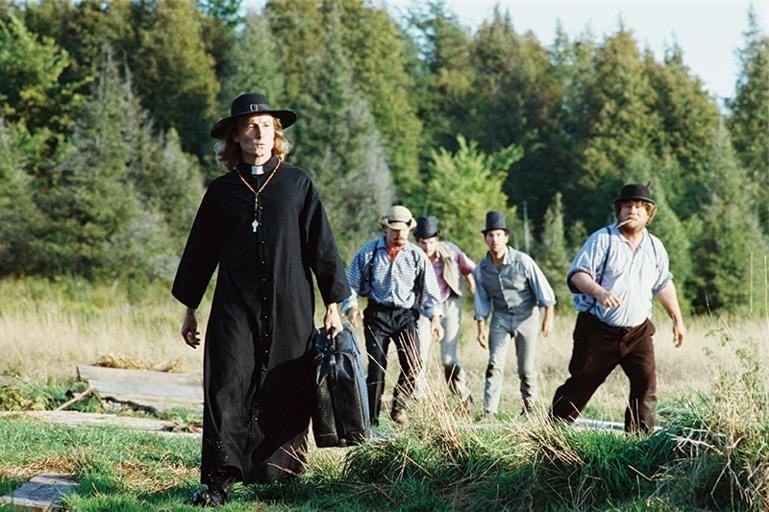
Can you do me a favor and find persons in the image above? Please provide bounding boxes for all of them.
[409,216,480,402]
[340,206,446,426]
[170,92,353,505]
[545,183,685,442]
[473,229,557,423]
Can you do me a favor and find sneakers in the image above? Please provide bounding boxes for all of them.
[190,482,230,509]
[389,406,411,428]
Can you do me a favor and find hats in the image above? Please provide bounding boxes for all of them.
[413,216,440,239]
[481,211,511,234]
[613,183,655,205]
[210,93,296,140]
[379,205,417,230]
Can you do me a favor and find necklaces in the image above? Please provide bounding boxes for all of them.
[237,158,282,232]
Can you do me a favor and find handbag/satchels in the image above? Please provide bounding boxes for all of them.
[358,271,371,297]
[312,322,370,449]
